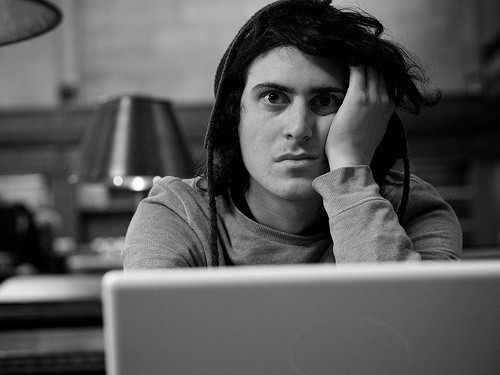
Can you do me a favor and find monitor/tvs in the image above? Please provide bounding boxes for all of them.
[102,265,500,375]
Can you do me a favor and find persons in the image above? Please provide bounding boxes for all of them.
[121,1,463,272]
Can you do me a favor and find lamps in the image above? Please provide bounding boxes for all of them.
[77,93,199,190]
